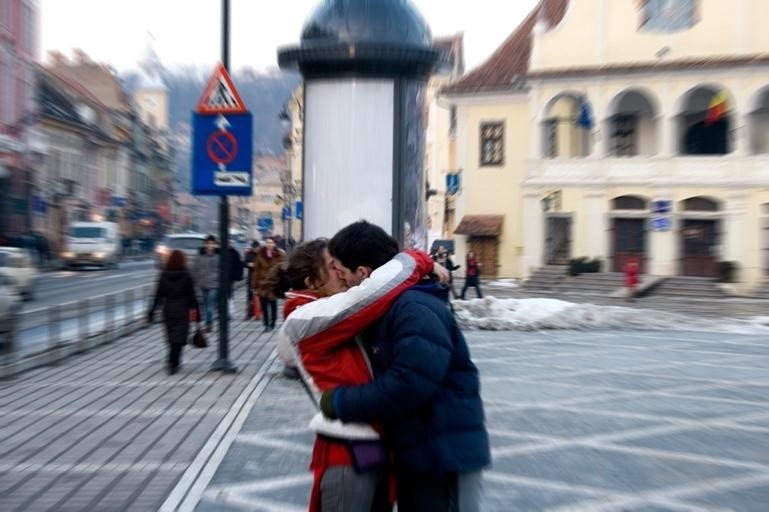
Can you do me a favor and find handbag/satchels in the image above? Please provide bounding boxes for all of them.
[192,321,210,349]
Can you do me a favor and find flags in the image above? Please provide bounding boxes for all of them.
[703,87,732,125]
[571,98,593,129]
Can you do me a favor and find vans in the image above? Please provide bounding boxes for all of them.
[59,222,122,272]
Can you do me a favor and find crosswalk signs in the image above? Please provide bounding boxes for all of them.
[195,64,249,117]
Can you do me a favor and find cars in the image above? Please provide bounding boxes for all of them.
[0,244,42,319]
[155,227,247,271]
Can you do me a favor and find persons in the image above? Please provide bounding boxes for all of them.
[262,235,450,512]
[315,220,493,512]
